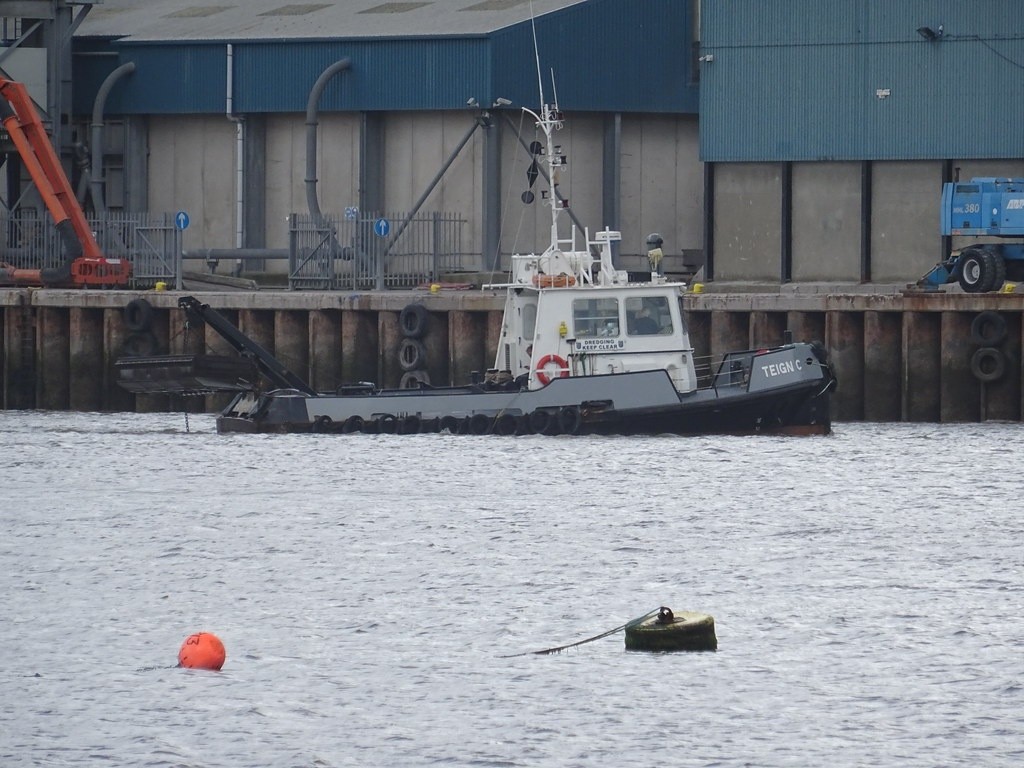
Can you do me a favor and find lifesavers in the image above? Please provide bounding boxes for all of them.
[533,275,575,287]
[309,405,582,437]
[969,347,1006,383]
[536,354,570,386]
[970,310,1009,348]
[395,303,429,391]
[124,297,157,356]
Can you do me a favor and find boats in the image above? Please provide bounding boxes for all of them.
[113,10,844,445]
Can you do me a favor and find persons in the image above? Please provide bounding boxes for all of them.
[632,307,664,335]
